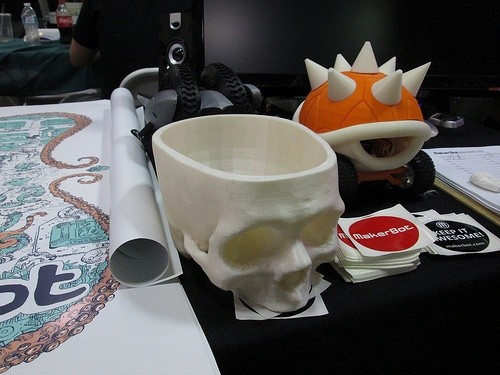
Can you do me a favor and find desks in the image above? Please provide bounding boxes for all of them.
[0,39,92,96]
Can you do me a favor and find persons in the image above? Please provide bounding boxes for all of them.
[69,0,204,99]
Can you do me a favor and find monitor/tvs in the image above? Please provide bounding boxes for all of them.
[193,0,500,98]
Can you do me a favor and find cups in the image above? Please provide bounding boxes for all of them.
[0,13,13,42]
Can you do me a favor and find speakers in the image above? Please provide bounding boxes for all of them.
[158,12,197,91]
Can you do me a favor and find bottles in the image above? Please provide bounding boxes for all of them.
[55,0,73,43]
[21,3,41,46]
